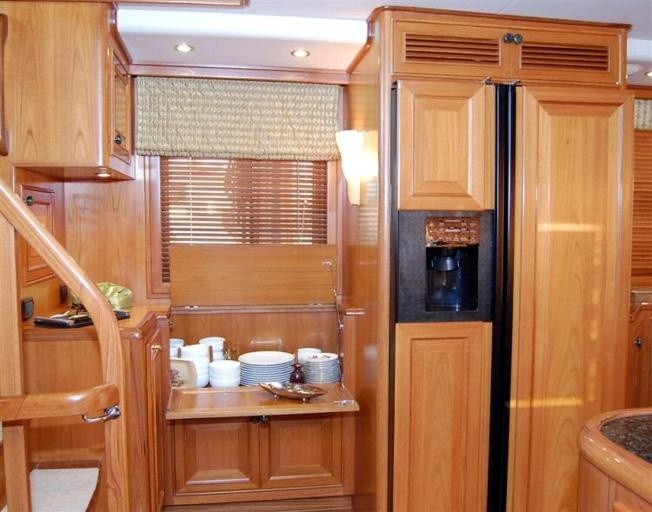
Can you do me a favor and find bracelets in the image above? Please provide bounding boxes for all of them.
[169,334,241,390]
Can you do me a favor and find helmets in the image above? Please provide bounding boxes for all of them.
[297,347,321,362]
[237,350,297,386]
[302,352,339,386]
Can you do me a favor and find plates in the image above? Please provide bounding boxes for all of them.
[28,317,164,512]
[175,417,347,493]
[5,1,136,179]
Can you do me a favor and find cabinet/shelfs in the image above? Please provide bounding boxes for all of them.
[175,417,347,493]
[28,317,164,512]
[289,363,307,384]
[5,1,136,179]
[347,34,626,510]
